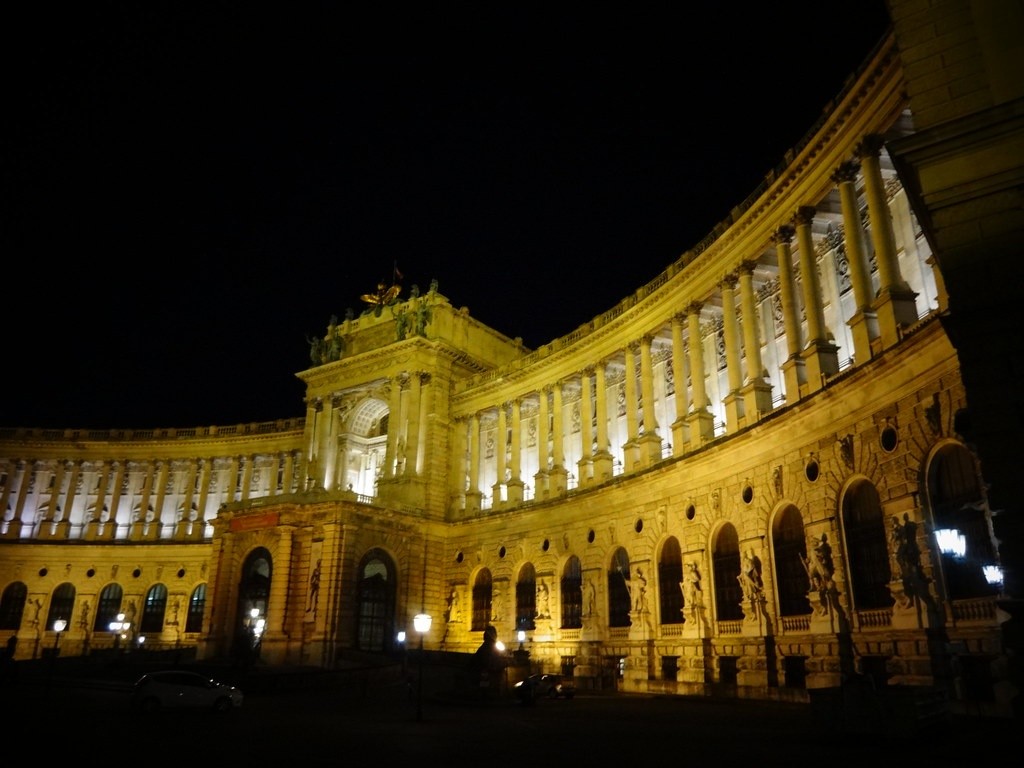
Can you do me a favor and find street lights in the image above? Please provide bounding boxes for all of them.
[108,612,136,651]
[53,617,67,649]
[413,613,432,722]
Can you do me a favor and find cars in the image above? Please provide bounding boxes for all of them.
[513,672,577,700]
[130,670,247,711]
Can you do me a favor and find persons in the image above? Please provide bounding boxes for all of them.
[888,516,914,579]
[681,561,699,608]
[491,590,504,620]
[448,592,461,622]
[77,600,91,627]
[307,557,321,613]
[805,537,831,591]
[627,570,649,612]
[121,600,135,627]
[327,330,342,363]
[394,310,409,341]
[581,579,595,615]
[309,336,324,369]
[28,598,41,623]
[736,551,760,604]
[535,584,549,619]
[415,304,428,339]
[166,594,180,625]
